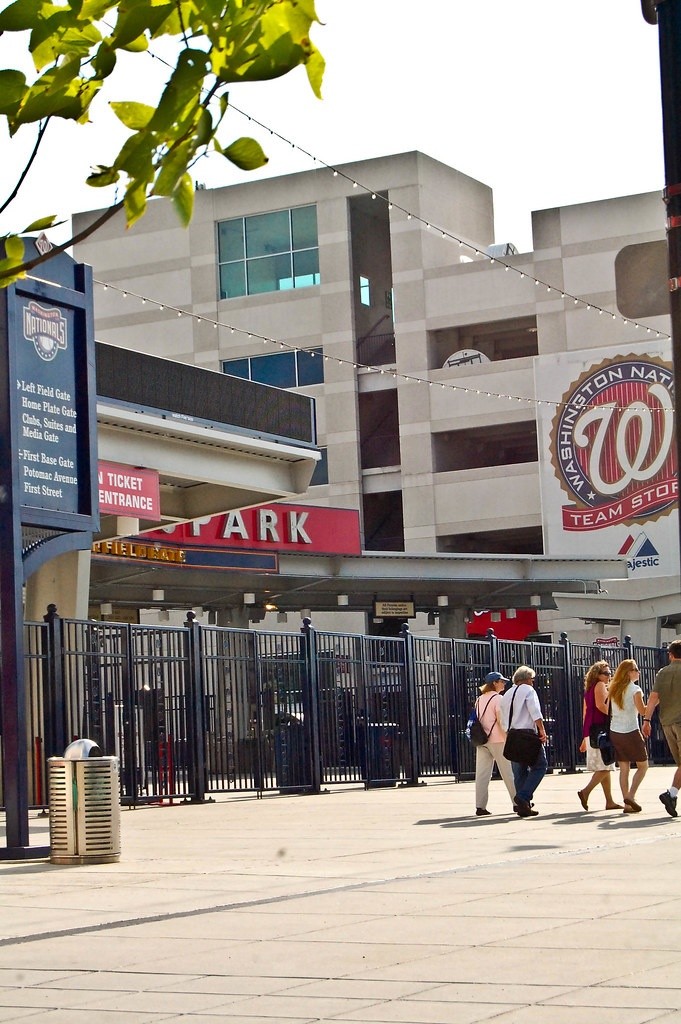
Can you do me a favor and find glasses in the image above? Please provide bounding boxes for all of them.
[629,667,639,673]
[598,671,612,676]
[496,680,506,684]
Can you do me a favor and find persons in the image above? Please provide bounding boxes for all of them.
[472,672,534,815]
[640,640,681,817]
[500,666,548,817]
[576,660,625,810]
[604,656,660,813]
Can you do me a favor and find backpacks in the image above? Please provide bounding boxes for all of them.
[465,694,498,745]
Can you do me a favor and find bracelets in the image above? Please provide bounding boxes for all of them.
[643,718,650,721]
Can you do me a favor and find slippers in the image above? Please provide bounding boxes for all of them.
[606,804,625,809]
[577,790,588,811]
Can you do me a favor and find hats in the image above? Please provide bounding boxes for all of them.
[485,672,511,683]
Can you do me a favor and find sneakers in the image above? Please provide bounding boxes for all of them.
[659,789,678,817]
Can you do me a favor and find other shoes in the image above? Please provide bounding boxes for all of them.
[623,795,642,812]
[624,807,639,813]
[513,803,535,812]
[476,807,491,815]
[517,802,539,817]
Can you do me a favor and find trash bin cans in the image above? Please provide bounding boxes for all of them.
[47,738,122,866]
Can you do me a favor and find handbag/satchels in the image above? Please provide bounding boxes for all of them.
[598,733,616,766]
[588,723,610,748]
[503,729,542,766]
